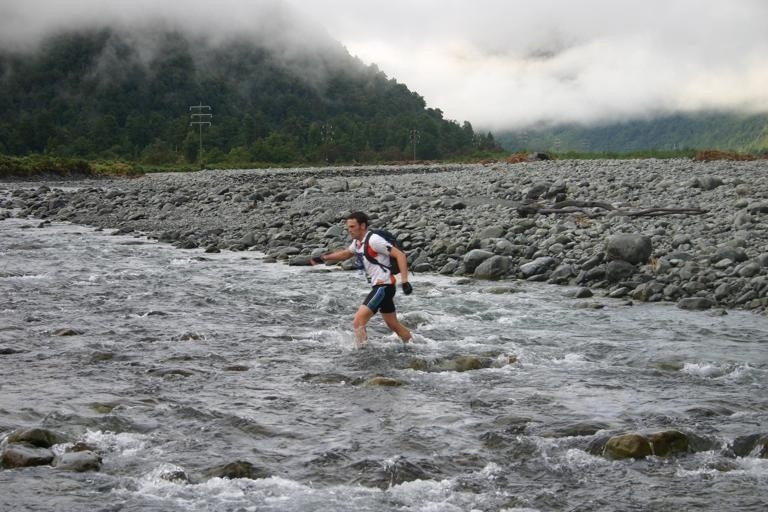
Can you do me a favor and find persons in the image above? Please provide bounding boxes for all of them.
[307,211,413,344]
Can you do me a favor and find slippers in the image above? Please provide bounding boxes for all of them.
[364,229,403,275]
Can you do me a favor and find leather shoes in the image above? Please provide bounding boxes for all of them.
[313,257,324,264]
[402,282,416,295]
